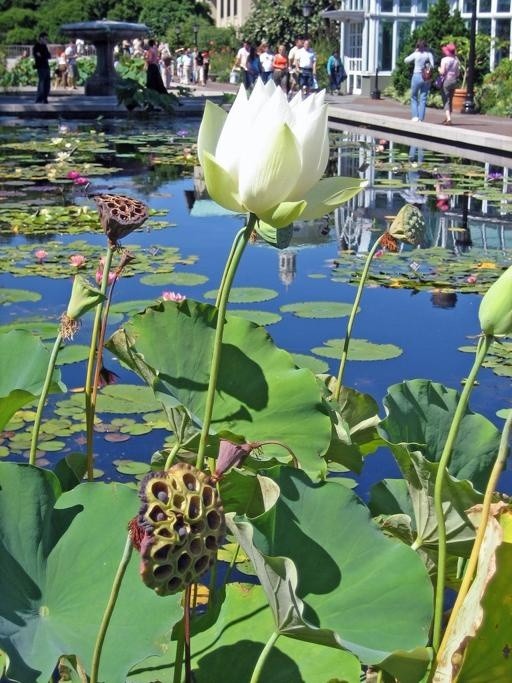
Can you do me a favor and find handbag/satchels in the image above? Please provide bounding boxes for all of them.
[432,74,445,89]
[310,76,318,90]
[422,62,433,80]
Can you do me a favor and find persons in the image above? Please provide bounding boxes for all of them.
[18,48,30,59]
[403,39,436,122]
[235,33,317,102]
[327,46,347,96]
[51,36,212,91]
[436,42,461,125]
[33,31,51,104]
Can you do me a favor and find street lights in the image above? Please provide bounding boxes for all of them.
[174,23,182,49]
[452,158,474,256]
[298,0,317,40]
[192,21,200,47]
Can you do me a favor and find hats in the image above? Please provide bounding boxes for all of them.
[441,44,455,56]
